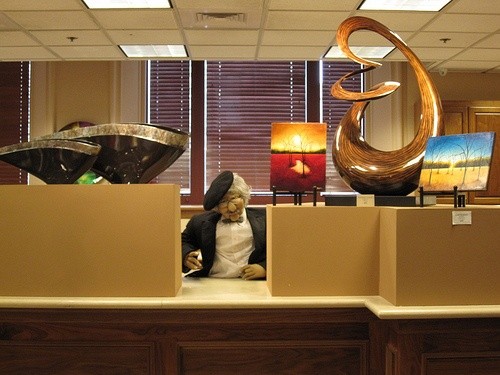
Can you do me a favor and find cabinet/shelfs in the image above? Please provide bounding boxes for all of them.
[414,100,500,205]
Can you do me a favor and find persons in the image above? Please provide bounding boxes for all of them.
[181,171,267,281]
